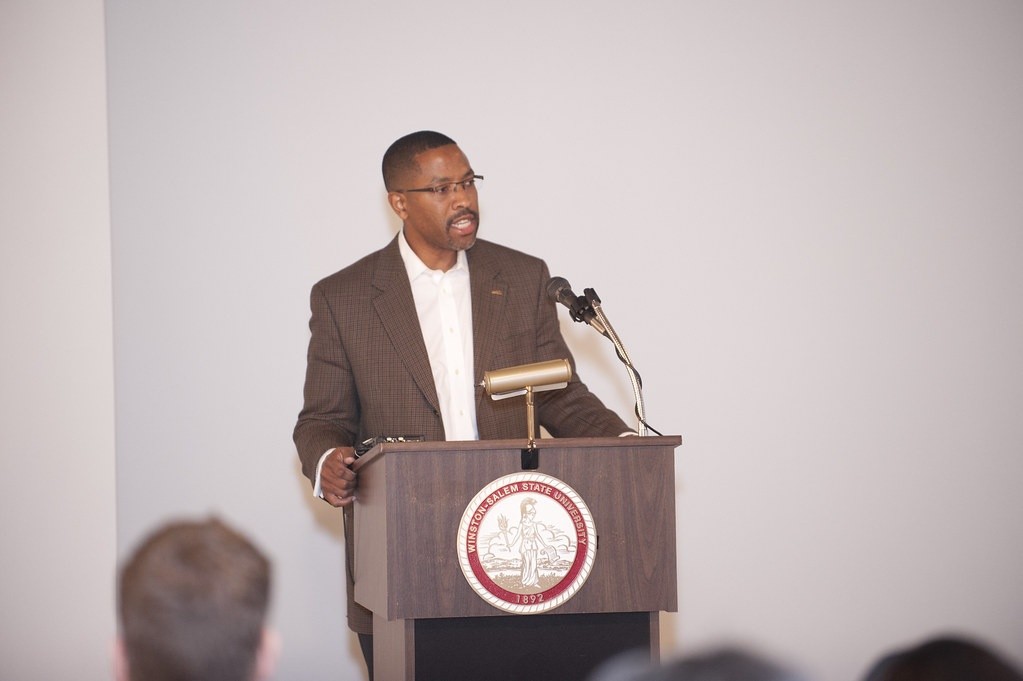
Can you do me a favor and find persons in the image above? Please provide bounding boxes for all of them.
[116,519,275,681]
[594,634,1023,681]
[293,130,638,681]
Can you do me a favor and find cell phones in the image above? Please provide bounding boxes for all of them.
[363,436,426,450]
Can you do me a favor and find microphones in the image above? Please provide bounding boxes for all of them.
[547,277,610,339]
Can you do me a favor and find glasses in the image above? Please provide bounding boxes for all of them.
[389,174,484,198]
